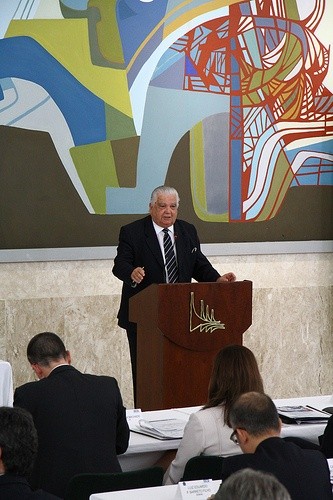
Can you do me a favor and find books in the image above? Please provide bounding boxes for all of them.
[126,408,190,440]
[275,404,332,425]
[174,479,221,500]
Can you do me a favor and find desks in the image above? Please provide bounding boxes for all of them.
[119,395,333,456]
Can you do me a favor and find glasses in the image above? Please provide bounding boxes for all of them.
[230,430,239,446]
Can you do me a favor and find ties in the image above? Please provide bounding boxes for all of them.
[162,228,179,284]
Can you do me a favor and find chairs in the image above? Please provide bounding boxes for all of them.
[72,466,164,500]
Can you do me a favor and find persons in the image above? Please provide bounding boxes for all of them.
[0,405,87,500]
[207,468,291,500]
[13,332,130,475]
[318,413,333,459]
[112,185,236,409]
[219,391,333,500]
[161,343,265,487]
